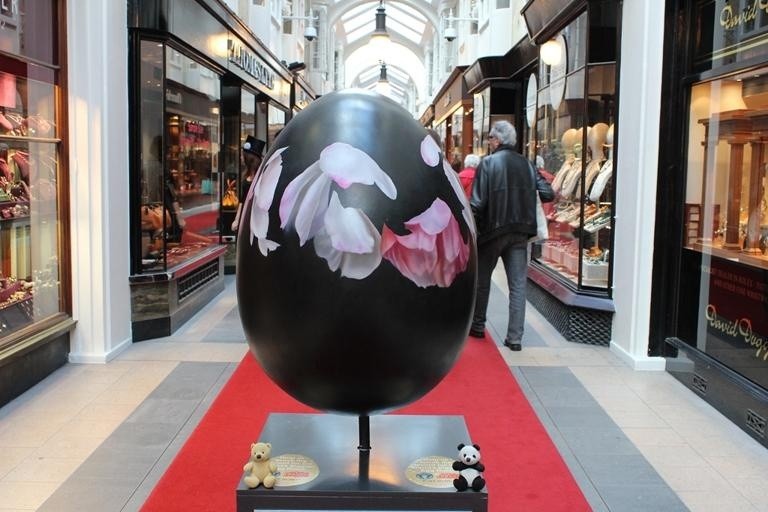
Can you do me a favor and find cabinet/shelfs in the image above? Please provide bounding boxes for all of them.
[0,57,65,358]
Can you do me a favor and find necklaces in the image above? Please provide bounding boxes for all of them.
[0,109,59,218]
[545,160,614,226]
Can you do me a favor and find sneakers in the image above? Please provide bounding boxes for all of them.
[503,339,521,351]
[469,328,485,338]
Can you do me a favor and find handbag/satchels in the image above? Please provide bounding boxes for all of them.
[142,206,172,231]
[531,189,549,244]
[222,179,241,209]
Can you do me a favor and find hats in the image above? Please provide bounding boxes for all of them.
[240,135,266,157]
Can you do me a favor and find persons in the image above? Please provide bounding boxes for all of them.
[147,136,185,246]
[458,153,481,200]
[469,120,554,352]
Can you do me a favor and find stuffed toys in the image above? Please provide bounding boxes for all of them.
[243,442,278,489]
[451,443,486,491]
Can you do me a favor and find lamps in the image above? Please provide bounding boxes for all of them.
[440,7,481,42]
[357,0,399,94]
[283,7,319,42]
